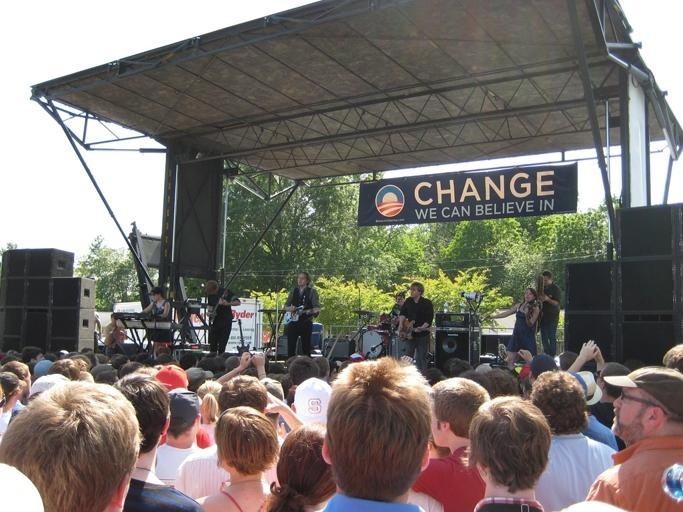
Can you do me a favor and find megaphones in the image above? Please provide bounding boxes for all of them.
[461,291,481,303]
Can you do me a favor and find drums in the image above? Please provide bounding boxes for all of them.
[355,331,384,359]
[378,329,389,348]
[368,325,377,331]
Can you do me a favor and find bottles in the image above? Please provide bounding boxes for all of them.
[443,302,448,313]
[661,463,683,502]
[460,301,465,312]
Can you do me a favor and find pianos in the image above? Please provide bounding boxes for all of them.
[111,313,168,322]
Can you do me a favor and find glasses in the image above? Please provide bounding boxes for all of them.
[619,392,669,417]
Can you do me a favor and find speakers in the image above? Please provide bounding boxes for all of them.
[0,248,95,353]
[435,312,481,368]
[324,336,355,358]
[565,202,683,368]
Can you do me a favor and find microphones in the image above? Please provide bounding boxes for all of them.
[146,292,154,296]
[371,340,384,351]
[293,305,304,312]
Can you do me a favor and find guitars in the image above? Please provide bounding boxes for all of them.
[207,291,228,318]
[398,318,433,338]
[284,305,319,325]
[237,319,249,356]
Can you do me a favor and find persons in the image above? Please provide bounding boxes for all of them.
[408,340,683,512]
[94,312,118,356]
[392,281,434,370]
[488,288,541,370]
[1,347,364,512]
[143,287,172,358]
[539,272,561,358]
[285,273,319,356]
[207,280,241,355]
[322,357,432,512]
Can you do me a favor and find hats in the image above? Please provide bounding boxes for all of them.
[155,364,213,423]
[295,376,334,437]
[603,366,683,418]
[29,374,70,397]
[576,371,602,406]
[33,360,53,376]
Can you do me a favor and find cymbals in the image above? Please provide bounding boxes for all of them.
[351,311,371,315]
